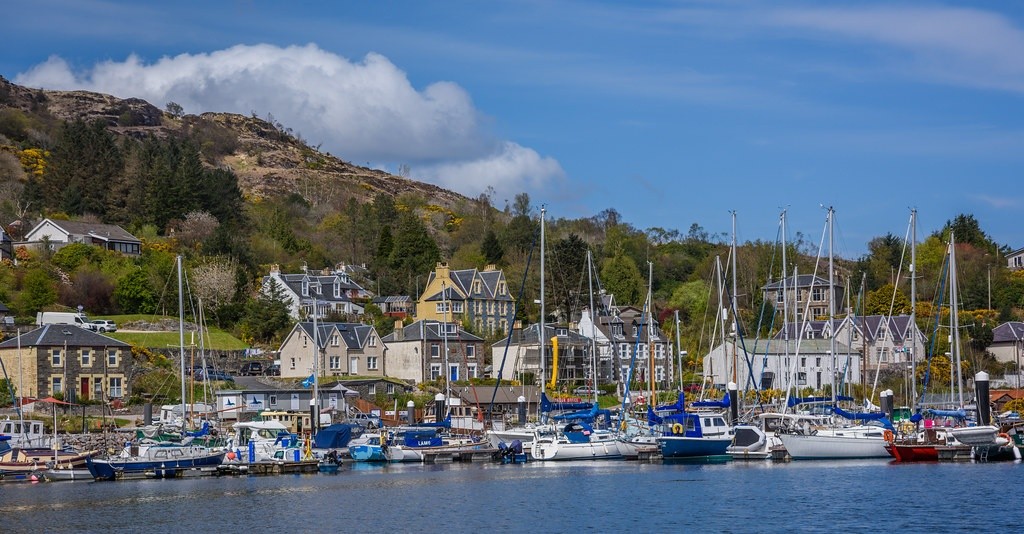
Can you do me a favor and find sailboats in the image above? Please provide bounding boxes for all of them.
[86,255,227,480]
[863,206,920,415]
[484,203,570,449]
[609,256,772,461]
[0,358,102,481]
[890,227,1024,444]
[724,206,892,463]
[529,249,638,462]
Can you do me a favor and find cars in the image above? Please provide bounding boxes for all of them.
[263,365,280,376]
[561,385,606,396]
[2,317,14,323]
[186,365,235,383]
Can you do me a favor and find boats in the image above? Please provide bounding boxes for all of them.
[883,428,971,463]
[306,281,501,464]
[973,433,1024,463]
[217,420,319,474]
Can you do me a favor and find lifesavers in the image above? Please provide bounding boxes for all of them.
[903,422,913,434]
[998,433,1010,446]
[637,396,644,405]
[673,423,683,434]
[856,419,860,423]
[884,430,893,442]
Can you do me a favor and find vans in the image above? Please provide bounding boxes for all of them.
[36,311,95,331]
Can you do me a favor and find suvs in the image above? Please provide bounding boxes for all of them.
[683,383,725,394]
[90,320,117,333]
[240,362,262,376]
